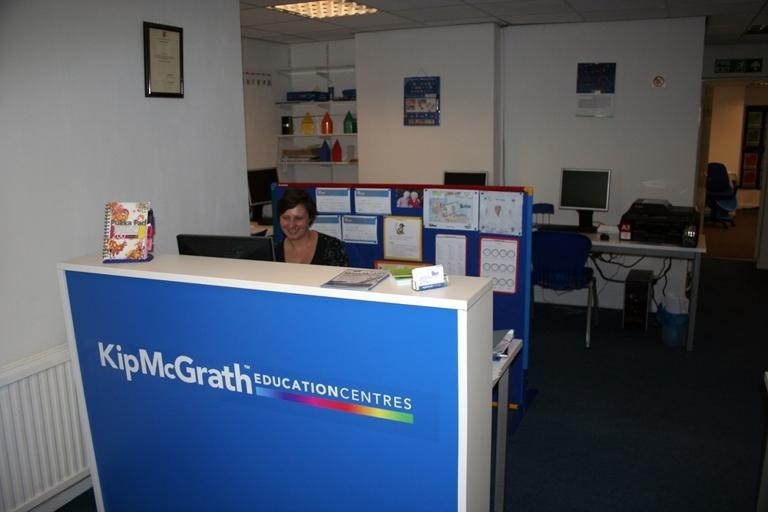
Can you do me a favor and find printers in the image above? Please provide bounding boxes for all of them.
[619,198,701,248]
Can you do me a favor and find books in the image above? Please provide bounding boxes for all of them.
[321,269,388,290]
[100,200,155,263]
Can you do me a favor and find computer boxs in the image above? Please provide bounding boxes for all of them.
[622,268,654,333]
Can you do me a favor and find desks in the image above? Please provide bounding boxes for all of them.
[580,224,707,352]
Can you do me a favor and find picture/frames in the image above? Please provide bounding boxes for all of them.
[142,21,185,99]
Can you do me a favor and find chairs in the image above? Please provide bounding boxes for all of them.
[530,229,599,349]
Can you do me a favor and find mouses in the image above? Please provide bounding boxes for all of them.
[600,232,610,240]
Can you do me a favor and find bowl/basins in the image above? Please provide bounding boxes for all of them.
[342,89,356,98]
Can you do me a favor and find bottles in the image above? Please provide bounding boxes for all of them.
[281,111,357,163]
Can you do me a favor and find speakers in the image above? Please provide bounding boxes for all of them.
[281,116,293,134]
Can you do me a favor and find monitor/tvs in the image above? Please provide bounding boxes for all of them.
[247,168,280,221]
[176,234,277,261]
[558,167,612,233]
[443,171,487,186]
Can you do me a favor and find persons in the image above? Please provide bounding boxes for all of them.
[268,189,347,267]
[396,190,423,210]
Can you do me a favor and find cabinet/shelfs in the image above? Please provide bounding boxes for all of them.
[274,97,359,166]
